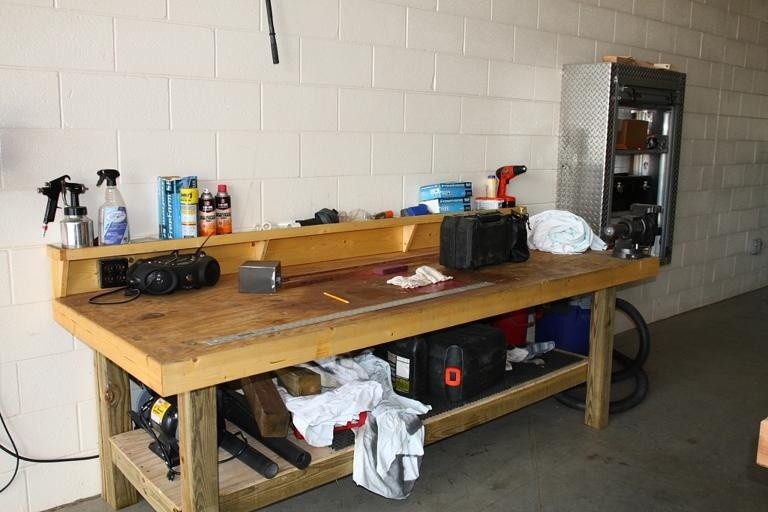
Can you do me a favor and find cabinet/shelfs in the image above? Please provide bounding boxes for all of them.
[555,62,686,266]
[47,206,660,512]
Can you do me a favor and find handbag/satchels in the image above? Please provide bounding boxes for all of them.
[436,211,528,270]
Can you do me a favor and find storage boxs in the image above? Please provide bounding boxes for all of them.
[428,324,507,402]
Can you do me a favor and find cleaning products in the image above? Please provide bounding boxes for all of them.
[96,168,131,246]
[34,175,95,248]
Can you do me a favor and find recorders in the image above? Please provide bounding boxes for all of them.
[126,253,222,296]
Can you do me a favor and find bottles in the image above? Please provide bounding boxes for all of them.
[180,184,232,239]
[487,175,498,198]
